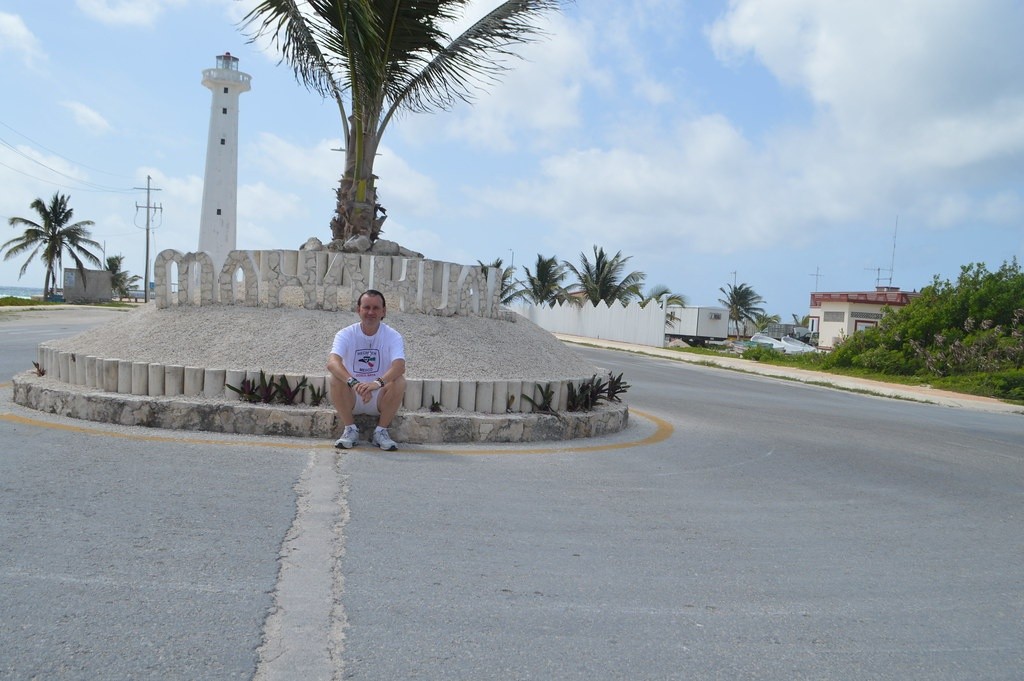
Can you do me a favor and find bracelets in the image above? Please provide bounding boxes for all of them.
[374,376,387,388]
[350,380,360,389]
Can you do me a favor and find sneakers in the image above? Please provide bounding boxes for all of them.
[371,428,399,451]
[334,426,359,449]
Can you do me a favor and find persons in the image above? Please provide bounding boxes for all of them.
[326,289,408,450]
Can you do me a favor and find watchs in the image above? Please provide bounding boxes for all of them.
[347,377,357,388]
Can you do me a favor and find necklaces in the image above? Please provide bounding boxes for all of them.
[360,321,380,357]
